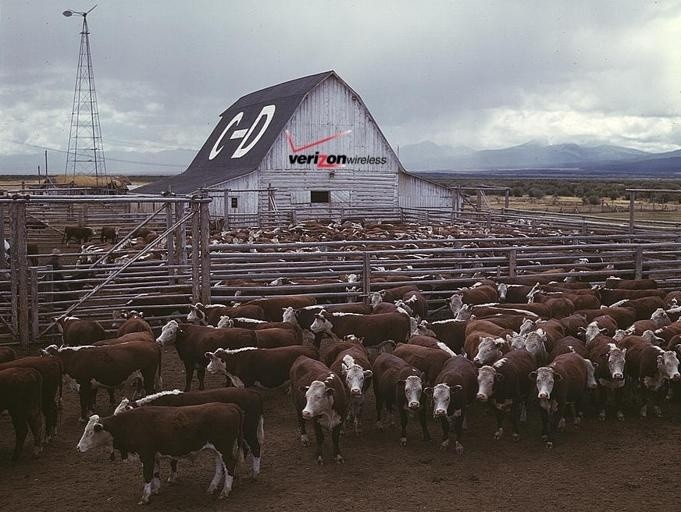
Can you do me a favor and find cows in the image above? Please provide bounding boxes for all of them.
[0,216,680,504]
